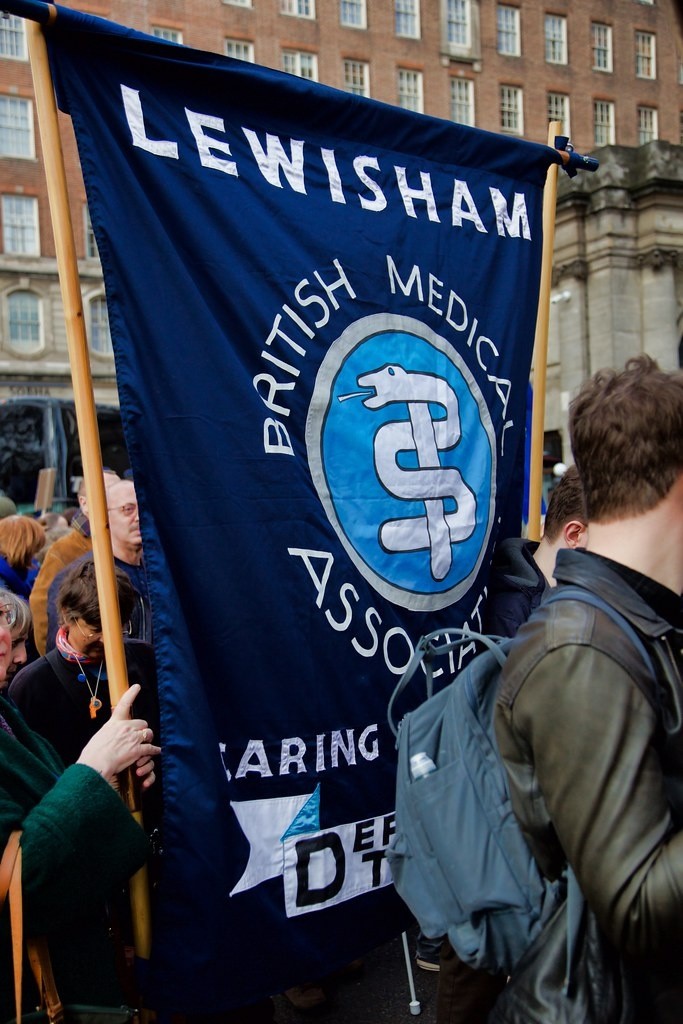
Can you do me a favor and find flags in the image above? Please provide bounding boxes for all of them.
[0,0,558,1019]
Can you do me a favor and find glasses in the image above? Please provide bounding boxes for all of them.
[0,603,18,629]
[72,614,132,641]
[105,503,135,514]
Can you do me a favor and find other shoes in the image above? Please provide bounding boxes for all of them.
[337,959,363,973]
[415,953,440,971]
[284,985,326,1009]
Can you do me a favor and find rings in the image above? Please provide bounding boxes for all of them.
[140,729,147,743]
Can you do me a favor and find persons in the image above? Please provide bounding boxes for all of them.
[0,353,683,1024]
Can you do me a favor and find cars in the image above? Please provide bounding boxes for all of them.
[1,396,129,504]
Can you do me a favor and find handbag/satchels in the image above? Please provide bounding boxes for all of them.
[485,873,637,1024]
[0,832,142,1024]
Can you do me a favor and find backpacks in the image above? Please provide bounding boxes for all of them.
[387,589,662,974]
